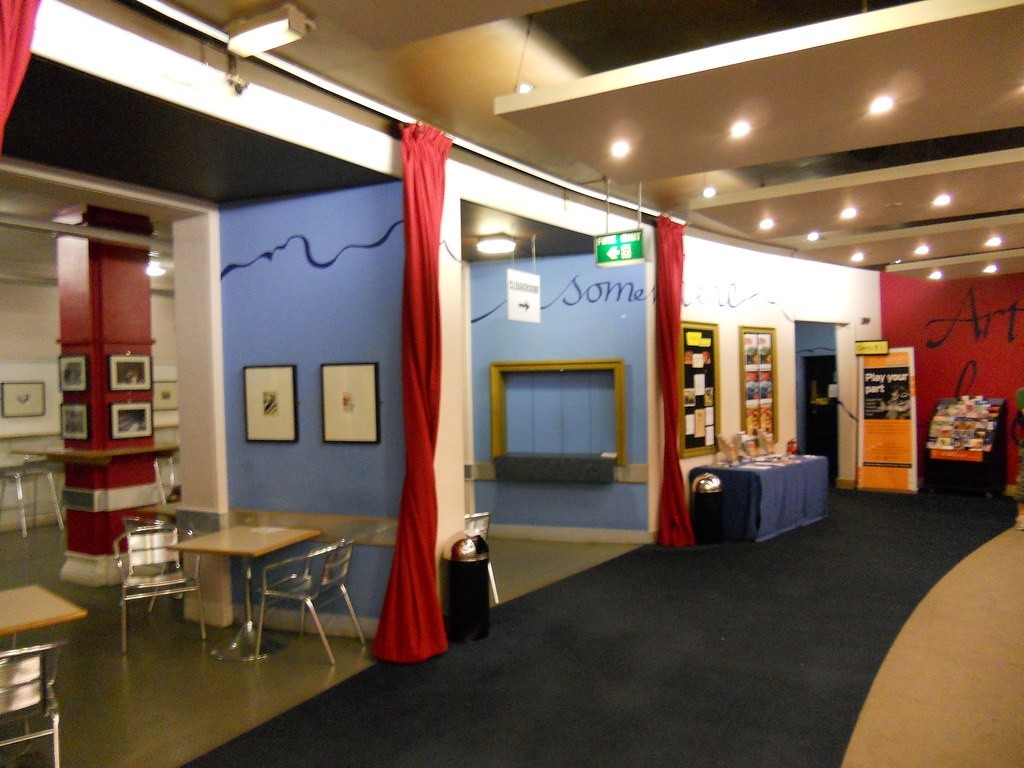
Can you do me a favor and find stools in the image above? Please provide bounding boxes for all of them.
[0,453,64,536]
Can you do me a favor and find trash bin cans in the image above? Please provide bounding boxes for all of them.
[441,531,493,644]
[692,473,726,548]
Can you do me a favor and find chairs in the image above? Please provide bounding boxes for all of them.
[112,517,206,655]
[464,512,500,604]
[0,640,70,768]
[255,536,366,667]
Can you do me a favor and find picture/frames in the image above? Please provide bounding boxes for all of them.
[1,383,46,417]
[60,403,89,440]
[109,401,154,441]
[153,381,178,411]
[243,364,300,443]
[58,354,88,393]
[738,326,779,446]
[677,321,722,458]
[108,353,154,392]
[320,363,381,444]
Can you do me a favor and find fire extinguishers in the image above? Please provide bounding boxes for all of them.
[788,439,798,453]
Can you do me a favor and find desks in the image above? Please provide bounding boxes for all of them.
[0,583,89,764]
[689,454,830,542]
[166,526,323,662]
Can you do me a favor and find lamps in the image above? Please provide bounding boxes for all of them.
[474,233,517,255]
[227,4,306,58]
